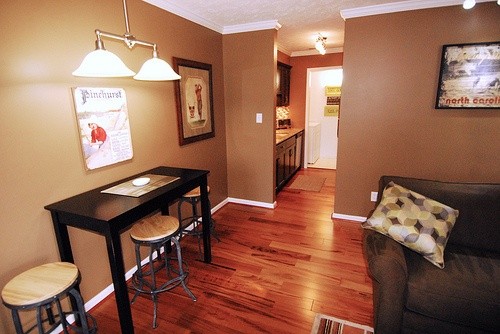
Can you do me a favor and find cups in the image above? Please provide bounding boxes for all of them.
[187,101,196,118]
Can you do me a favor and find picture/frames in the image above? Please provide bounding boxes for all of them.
[172,56,215,146]
[435,41,500,109]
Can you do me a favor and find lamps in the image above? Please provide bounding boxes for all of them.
[315,33,327,55]
[71,0,182,81]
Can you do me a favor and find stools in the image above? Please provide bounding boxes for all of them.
[178,185,220,256]
[130,216,197,328]
[2,262,97,334]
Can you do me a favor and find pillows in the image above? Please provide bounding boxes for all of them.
[361,181,459,269]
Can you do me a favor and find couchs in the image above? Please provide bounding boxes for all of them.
[364,176,500,334]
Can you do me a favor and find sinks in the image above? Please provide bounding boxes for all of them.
[276,133,288,142]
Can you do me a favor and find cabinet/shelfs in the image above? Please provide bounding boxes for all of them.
[276,61,304,194]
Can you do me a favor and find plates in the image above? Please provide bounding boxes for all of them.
[132,176,151,186]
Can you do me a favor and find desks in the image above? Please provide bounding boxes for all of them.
[43,166,210,334]
